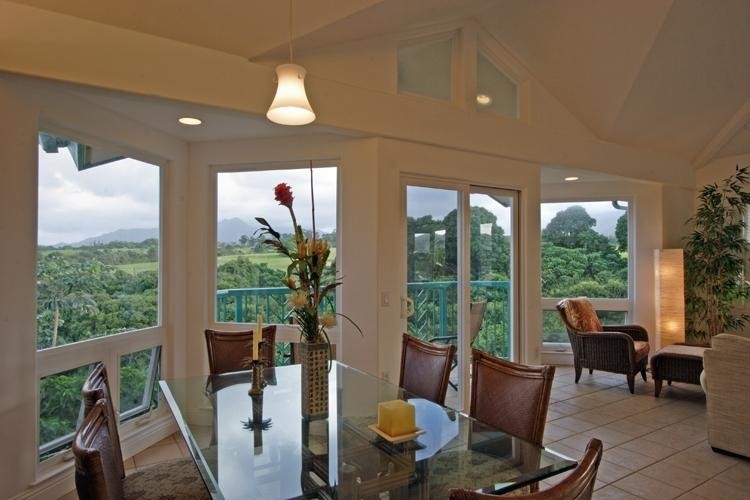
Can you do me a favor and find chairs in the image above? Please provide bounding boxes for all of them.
[204,323,280,446]
[447,438,603,500]
[700,334,750,463]
[72,362,213,499]
[415,347,557,499]
[342,332,455,457]
[556,296,650,394]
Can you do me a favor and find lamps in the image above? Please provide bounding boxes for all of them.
[266,0,317,125]
[654,249,686,351]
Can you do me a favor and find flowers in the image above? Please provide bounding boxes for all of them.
[253,182,363,372]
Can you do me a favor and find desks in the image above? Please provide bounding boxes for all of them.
[157,359,579,499]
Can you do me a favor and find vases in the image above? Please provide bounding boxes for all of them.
[299,342,330,420]
